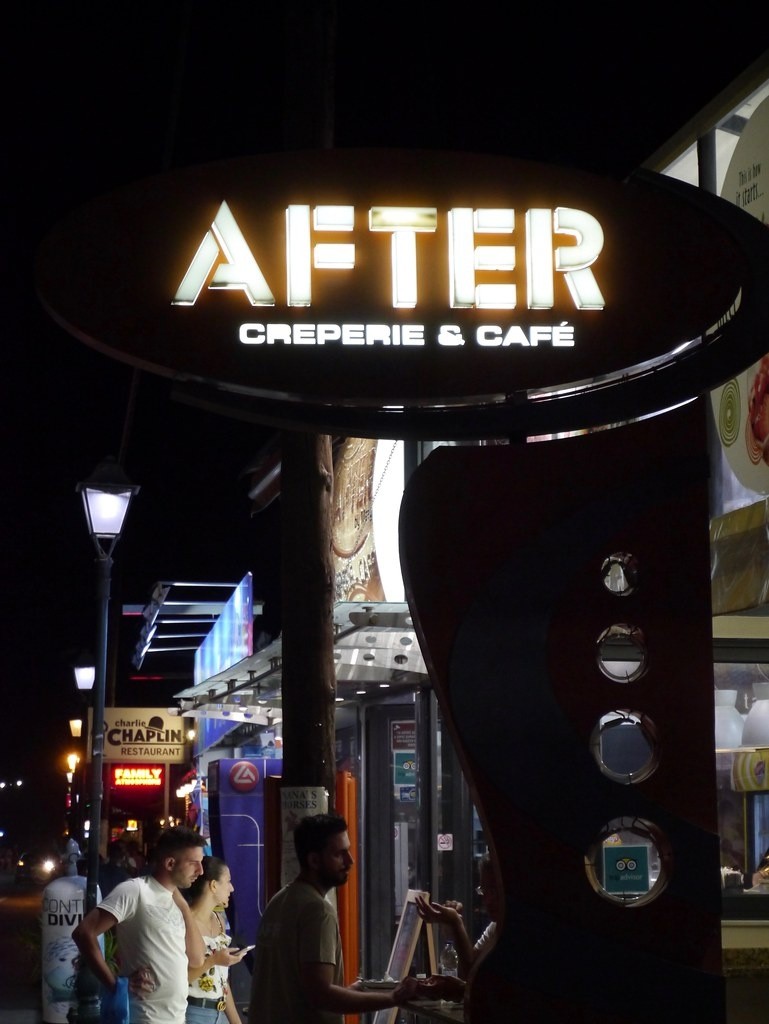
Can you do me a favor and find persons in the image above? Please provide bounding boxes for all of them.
[248,815,419,1024]
[414,854,499,1002]
[71,827,247,1024]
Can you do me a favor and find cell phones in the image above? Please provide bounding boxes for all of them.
[233,945,256,955]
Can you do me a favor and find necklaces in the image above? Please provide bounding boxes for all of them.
[195,911,215,939]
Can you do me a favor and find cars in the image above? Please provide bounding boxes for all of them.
[13,853,49,885]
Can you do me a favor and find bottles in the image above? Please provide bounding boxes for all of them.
[440,938,458,979]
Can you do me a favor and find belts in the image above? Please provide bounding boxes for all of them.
[187,995,226,1011]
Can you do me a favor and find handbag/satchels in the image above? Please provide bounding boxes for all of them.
[101,977,130,1024]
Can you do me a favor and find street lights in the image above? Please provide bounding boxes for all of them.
[67,752,80,839]
[73,454,144,1023]
[72,651,96,873]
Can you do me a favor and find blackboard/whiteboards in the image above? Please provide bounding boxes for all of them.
[369,889,437,1024]
